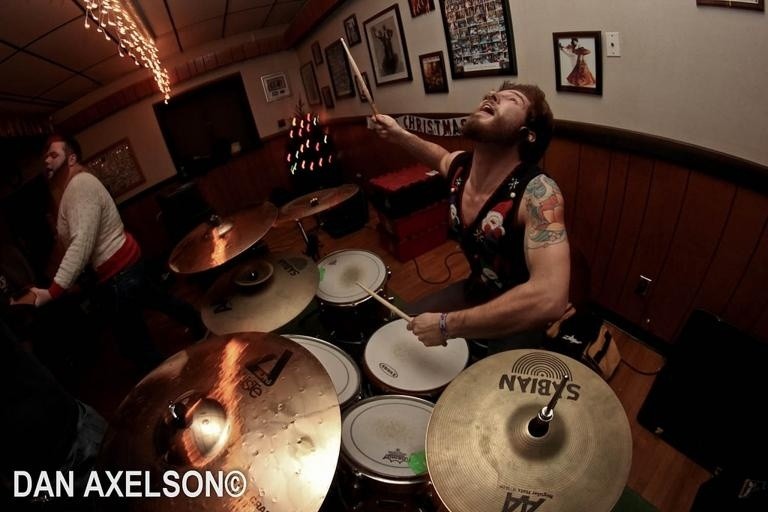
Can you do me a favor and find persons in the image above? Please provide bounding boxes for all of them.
[372,25,398,75]
[28,135,210,376]
[369,80,571,361]
[1,290,107,467]
[558,37,596,87]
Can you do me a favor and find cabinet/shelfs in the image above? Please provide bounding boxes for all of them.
[367,163,450,263]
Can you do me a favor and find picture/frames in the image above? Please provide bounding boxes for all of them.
[81,136,148,200]
[297,1,605,111]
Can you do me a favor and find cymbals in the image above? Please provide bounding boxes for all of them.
[425,348,633,512]
[99,332,342,512]
[169,201,278,275]
[280,183,359,218]
[200,251,320,337]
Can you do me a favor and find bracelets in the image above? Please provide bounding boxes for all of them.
[439,311,448,347]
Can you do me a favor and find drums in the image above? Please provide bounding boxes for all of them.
[317,248,394,345]
[359,314,469,405]
[277,334,363,415]
[340,394,436,512]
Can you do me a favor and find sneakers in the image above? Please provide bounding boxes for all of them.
[189,311,205,336]
